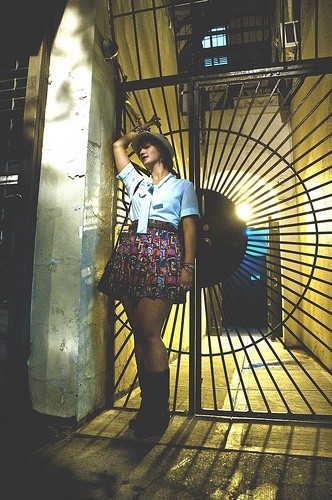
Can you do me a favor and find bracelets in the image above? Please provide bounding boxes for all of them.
[129,132,135,139]
[133,126,143,133]
[183,266,193,275]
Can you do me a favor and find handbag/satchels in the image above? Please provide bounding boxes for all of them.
[97,249,119,300]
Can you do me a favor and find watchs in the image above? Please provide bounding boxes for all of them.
[183,263,195,269]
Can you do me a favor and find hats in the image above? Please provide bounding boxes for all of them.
[132,132,174,161]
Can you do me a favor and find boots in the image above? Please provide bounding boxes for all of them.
[141,368,170,437]
[129,352,147,429]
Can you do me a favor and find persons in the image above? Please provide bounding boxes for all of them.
[98,114,201,440]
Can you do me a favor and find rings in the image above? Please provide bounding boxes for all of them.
[183,286,187,289]
[181,285,184,287]
[187,285,191,288]
